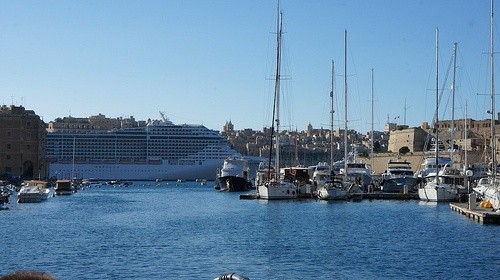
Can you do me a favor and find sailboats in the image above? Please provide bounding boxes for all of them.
[256,1,500,208]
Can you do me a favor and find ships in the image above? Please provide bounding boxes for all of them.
[46,123,270,182]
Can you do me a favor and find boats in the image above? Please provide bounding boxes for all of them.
[0,177,119,210]
[213,153,252,192]
